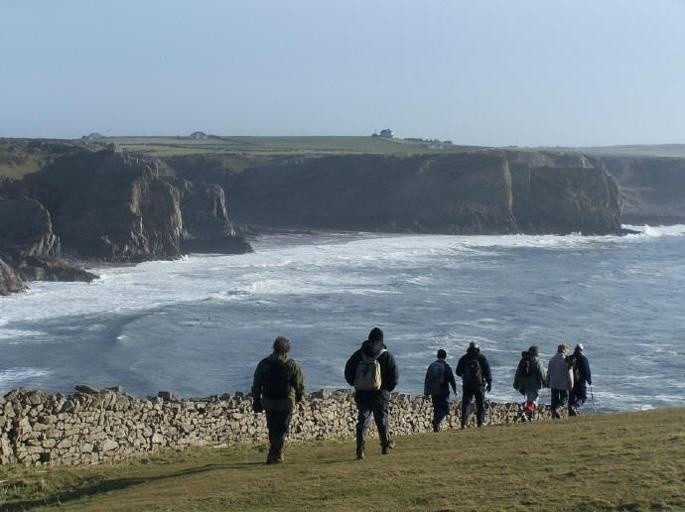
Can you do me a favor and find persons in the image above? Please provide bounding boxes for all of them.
[545,344,572,420]
[513,345,547,422]
[252,335,304,465]
[567,343,592,418]
[422,349,458,433]
[344,327,400,460]
[455,341,493,429]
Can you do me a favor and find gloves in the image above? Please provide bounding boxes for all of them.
[543,384,550,388]
[588,381,592,385]
[454,391,457,397]
[296,395,304,402]
[253,399,264,413]
[486,382,492,392]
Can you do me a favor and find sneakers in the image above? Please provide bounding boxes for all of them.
[521,406,581,421]
[358,452,366,459]
[383,440,395,454]
[266,452,284,464]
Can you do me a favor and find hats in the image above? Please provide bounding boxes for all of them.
[368,327,382,341]
[437,350,446,357]
[470,341,479,348]
[576,343,583,353]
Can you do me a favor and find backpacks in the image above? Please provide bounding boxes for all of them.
[353,347,387,392]
[462,357,483,385]
[426,360,447,396]
[521,354,533,377]
[567,355,580,383]
[264,360,293,401]
